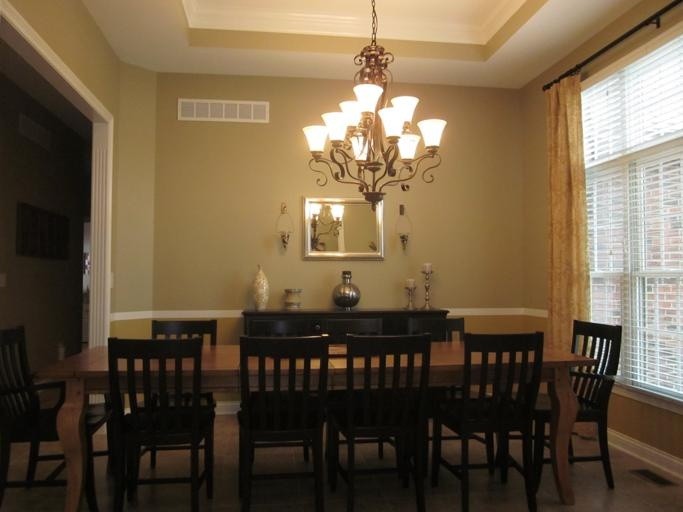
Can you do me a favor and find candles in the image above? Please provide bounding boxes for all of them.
[423,262,432,273]
[406,278,415,287]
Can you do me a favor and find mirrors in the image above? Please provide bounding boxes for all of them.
[300,195,385,261]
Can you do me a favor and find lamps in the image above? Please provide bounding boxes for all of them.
[308,203,345,251]
[301,1,448,215]
[275,202,294,251]
[392,204,413,253]
